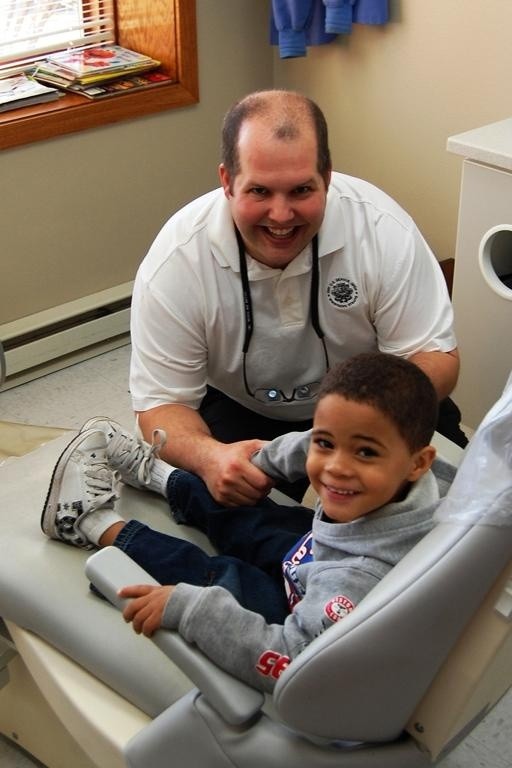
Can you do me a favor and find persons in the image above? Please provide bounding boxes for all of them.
[127,87,462,509]
[36,350,444,750]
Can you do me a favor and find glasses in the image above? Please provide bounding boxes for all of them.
[243,338,331,405]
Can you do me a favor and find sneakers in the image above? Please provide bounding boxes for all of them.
[41,429,116,550]
[80,415,163,492]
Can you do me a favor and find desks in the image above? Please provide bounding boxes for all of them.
[445,117,512,430]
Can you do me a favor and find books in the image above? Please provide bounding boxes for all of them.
[0,43,171,114]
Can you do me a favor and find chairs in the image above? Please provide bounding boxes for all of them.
[0,370,512,768]
